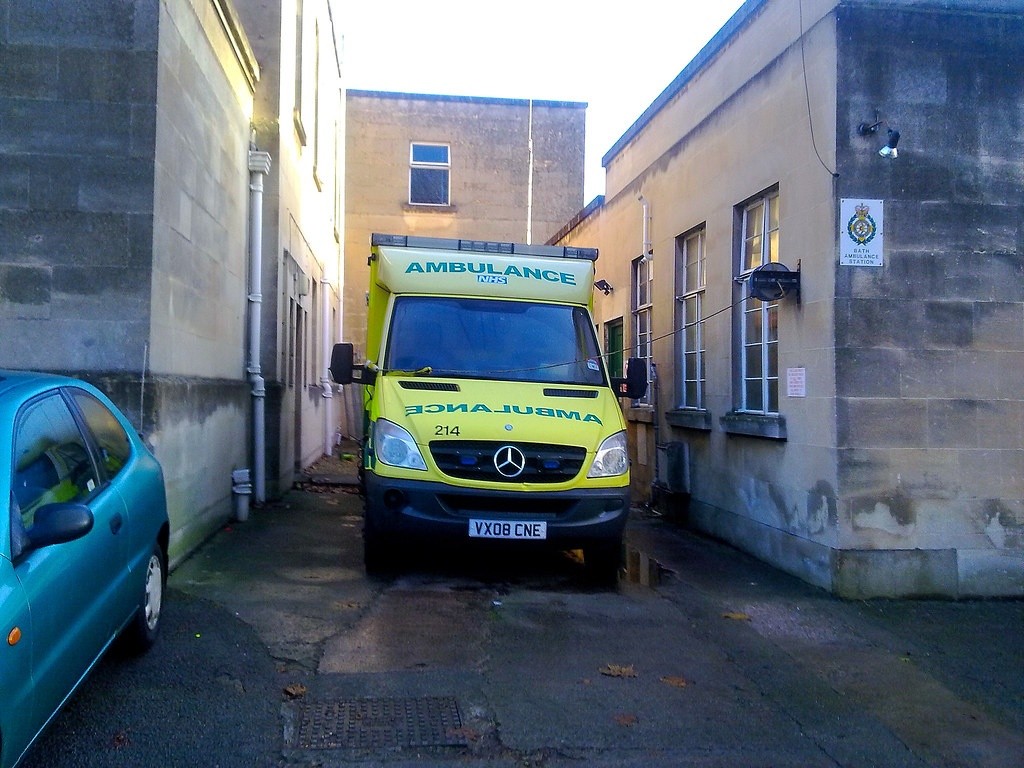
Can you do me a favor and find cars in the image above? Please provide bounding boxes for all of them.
[0,369,171,768]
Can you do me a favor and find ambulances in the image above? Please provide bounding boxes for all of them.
[329,232,653,583]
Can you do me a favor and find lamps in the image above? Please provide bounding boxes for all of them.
[857,107,901,159]
[594,280,613,296]
